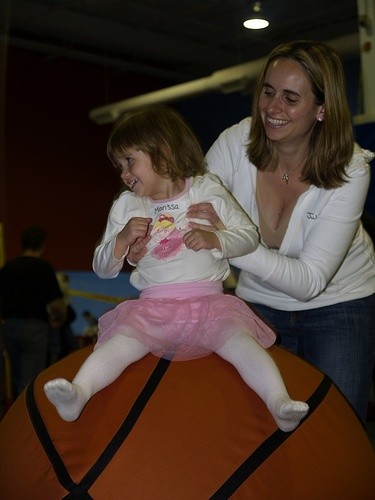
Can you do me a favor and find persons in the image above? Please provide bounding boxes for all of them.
[43,108,309,433]
[0,225,70,404]
[126,41,375,428]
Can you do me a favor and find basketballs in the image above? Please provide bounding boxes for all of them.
[0,341,374,500]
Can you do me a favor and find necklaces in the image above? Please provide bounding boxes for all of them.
[271,147,310,185]
